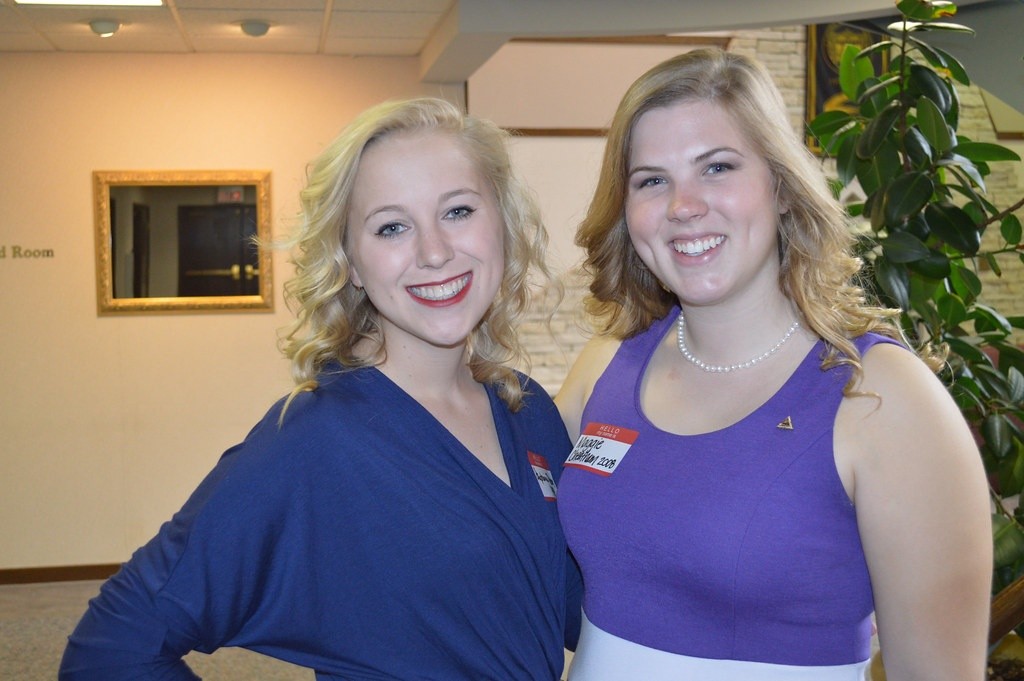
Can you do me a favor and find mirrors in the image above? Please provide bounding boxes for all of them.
[92,168,277,317]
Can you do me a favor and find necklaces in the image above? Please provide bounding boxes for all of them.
[676,311,800,374]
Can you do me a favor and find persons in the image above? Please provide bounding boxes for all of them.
[554,47,993,681]
[57,96,583,681]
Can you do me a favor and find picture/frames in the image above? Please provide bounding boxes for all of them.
[806,20,893,159]
